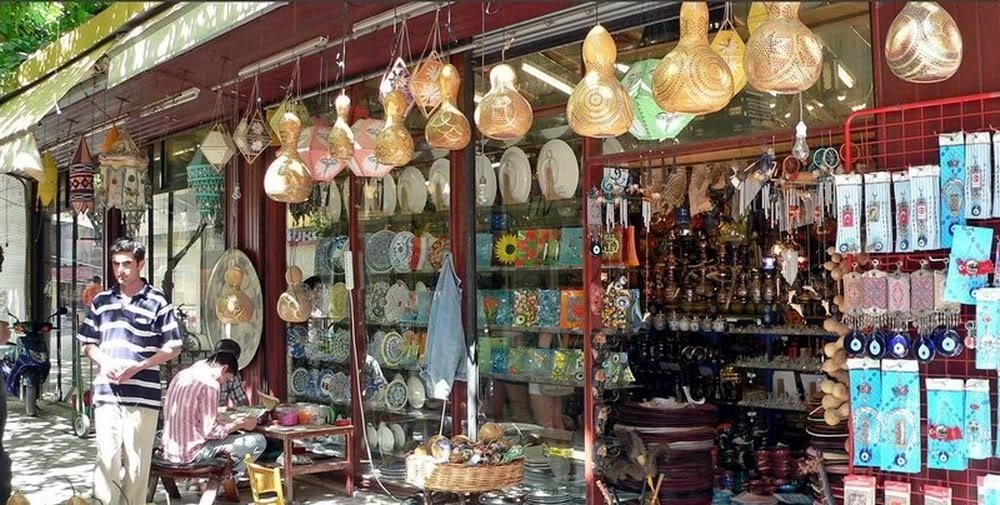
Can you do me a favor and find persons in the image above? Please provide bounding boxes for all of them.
[473,269,540,446]
[0,244,23,505]
[75,236,182,505]
[517,267,573,450]
[291,273,331,403]
[79,274,105,313]
[208,337,253,410]
[318,318,389,408]
[160,350,268,505]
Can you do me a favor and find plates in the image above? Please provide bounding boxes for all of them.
[286,233,452,483]
[315,137,580,224]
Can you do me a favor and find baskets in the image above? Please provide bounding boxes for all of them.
[406,456,524,493]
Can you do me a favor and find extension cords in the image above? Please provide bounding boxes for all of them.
[343,251,354,291]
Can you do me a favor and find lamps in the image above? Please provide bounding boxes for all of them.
[237,0,458,80]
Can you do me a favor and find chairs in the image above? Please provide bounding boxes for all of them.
[243,453,285,505]
[146,446,241,505]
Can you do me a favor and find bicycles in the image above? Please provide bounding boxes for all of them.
[173,301,202,359]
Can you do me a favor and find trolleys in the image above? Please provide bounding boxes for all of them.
[71,314,99,438]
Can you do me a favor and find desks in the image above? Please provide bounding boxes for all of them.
[254,424,354,504]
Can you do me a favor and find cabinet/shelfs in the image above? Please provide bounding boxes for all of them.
[286,197,583,464]
[716,326,837,415]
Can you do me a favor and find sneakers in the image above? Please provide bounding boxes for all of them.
[187,475,239,496]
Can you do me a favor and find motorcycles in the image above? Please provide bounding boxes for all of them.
[1,289,70,417]
